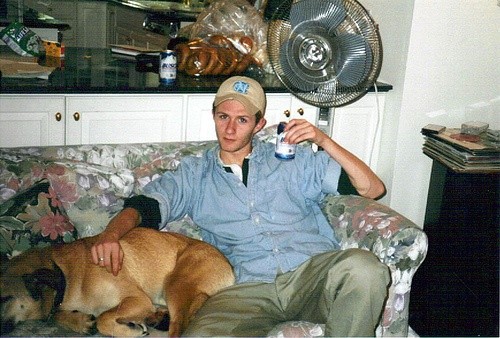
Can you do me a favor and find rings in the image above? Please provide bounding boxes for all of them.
[98,257,104,261]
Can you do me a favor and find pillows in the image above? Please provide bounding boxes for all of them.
[0,174,83,262]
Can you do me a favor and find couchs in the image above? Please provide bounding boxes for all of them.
[0,122,428,338]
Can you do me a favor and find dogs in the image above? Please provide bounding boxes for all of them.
[0,226,234,338]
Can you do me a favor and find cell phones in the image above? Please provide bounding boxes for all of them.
[422,124,445,135]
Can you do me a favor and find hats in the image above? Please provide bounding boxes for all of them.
[214,76,266,117]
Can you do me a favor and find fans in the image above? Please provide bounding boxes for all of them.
[264,0,384,152]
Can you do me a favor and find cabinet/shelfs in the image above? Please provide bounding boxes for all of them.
[185,91,387,174]
[0,94,186,149]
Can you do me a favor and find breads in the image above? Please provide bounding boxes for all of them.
[176,32,265,76]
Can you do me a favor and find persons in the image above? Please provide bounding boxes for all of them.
[92,75,391,338]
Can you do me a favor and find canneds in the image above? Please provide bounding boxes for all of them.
[275,122,297,160]
[159,49,177,83]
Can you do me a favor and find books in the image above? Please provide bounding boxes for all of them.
[420,125,500,173]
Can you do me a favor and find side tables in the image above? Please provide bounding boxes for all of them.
[400,127,499,337]
